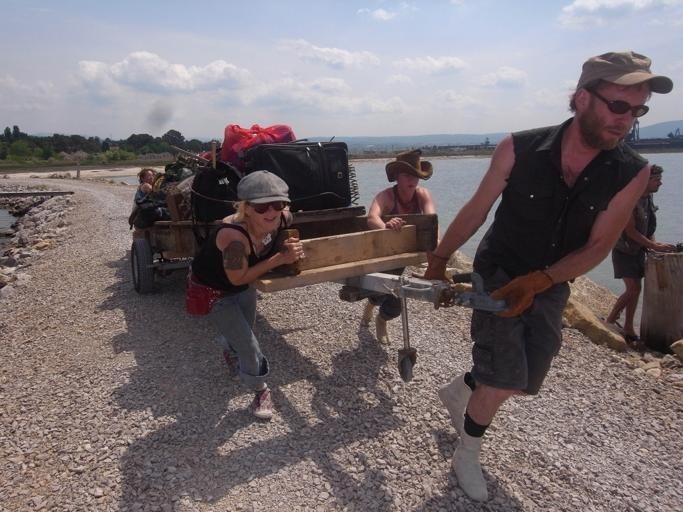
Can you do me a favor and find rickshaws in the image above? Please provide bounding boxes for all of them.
[132,214,506,382]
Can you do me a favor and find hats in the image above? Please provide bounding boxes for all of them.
[385,150,433,182]
[236,170,292,204]
[577,50,673,94]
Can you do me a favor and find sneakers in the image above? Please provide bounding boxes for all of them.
[252,383,272,419]
[365,300,391,344]
[223,349,241,381]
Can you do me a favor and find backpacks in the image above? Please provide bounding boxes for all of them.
[191,160,242,222]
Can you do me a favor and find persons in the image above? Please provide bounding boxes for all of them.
[360,148,434,345]
[600,164,676,342]
[189,170,306,419]
[423,51,672,502]
[130,168,172,229]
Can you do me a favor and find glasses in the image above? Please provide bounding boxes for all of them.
[248,202,287,214]
[593,91,649,117]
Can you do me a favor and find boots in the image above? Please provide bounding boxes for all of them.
[438,372,492,502]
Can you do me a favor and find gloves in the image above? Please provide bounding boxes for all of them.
[489,270,554,318]
[424,251,454,284]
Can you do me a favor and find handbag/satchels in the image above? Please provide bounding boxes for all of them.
[184,280,224,315]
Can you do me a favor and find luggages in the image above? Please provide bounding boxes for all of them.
[244,142,351,212]
